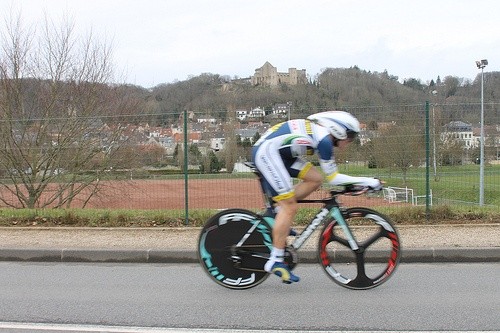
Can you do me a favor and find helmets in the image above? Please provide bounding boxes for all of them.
[307,110,361,140]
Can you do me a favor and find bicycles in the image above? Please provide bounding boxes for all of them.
[197,161,402,292]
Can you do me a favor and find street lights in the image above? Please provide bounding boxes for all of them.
[475,59,488,206]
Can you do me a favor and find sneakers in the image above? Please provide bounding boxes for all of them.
[263,259,299,283]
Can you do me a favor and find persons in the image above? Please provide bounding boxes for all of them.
[251,110,383,284]
